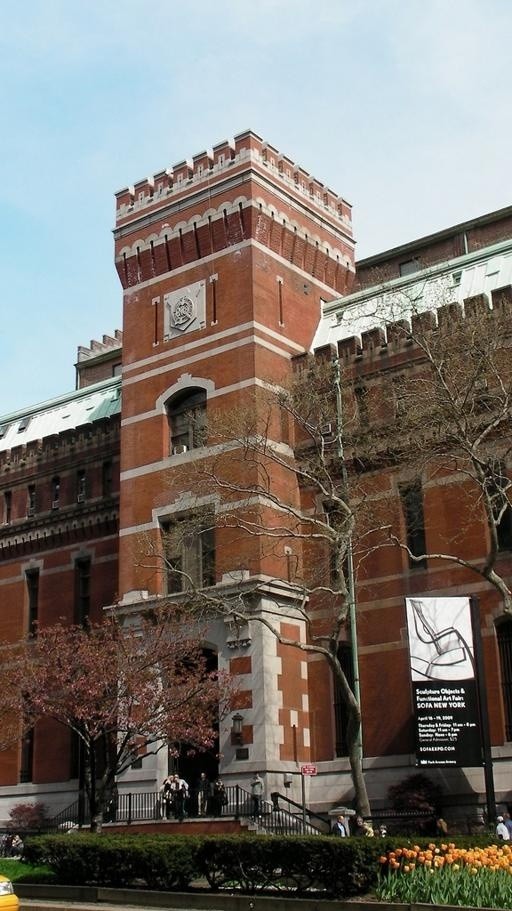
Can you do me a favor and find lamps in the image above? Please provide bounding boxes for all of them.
[230,713,245,745]
[128,726,138,750]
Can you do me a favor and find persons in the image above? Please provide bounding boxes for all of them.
[1,833,25,858]
[352,817,367,837]
[494,817,509,841]
[157,772,226,819]
[107,788,121,825]
[373,830,380,838]
[379,825,389,837]
[501,813,511,839]
[331,816,349,839]
[250,773,264,820]
[435,819,448,838]
